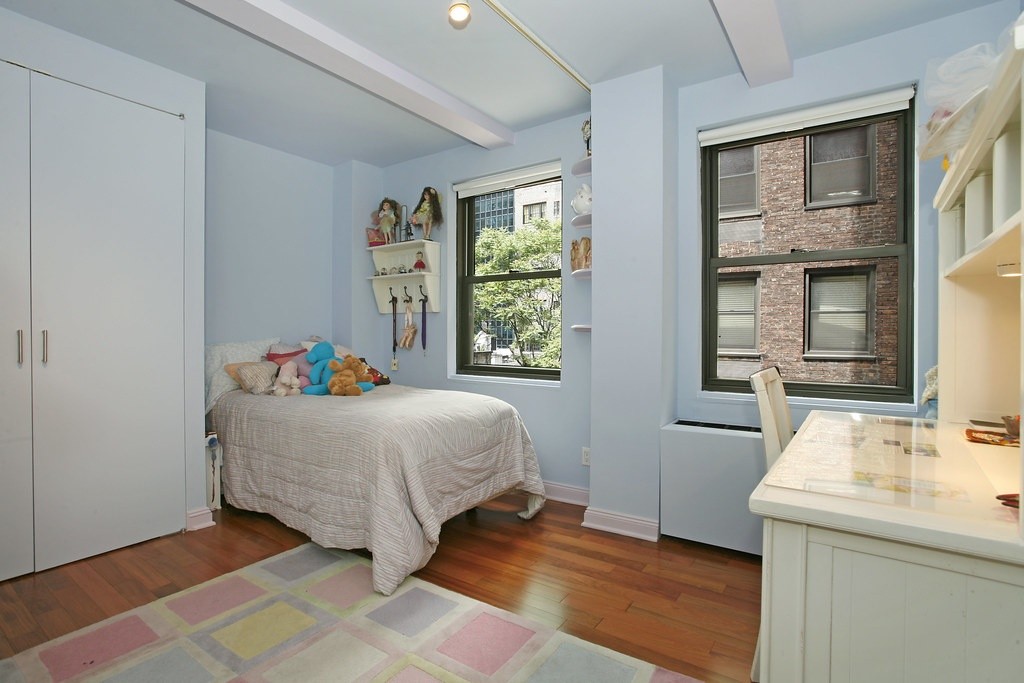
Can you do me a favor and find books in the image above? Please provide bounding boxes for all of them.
[968,420,1007,433]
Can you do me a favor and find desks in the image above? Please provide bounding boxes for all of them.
[748,411,1024,683]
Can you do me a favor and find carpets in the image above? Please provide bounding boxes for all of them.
[0,542,711,683]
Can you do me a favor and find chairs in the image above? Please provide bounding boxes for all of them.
[750,365,795,682]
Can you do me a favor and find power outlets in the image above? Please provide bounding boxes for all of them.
[581,447,590,466]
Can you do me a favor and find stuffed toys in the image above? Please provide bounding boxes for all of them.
[273,340,374,395]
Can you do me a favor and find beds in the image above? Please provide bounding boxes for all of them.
[205,382,547,597]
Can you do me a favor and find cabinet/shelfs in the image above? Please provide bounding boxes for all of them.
[933,24,1024,551]
[0,5,215,585]
[365,239,442,313]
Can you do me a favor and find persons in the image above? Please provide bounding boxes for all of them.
[413,187,441,240]
[375,197,399,245]
[413,251,426,272]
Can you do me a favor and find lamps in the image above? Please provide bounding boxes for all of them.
[448,0,471,22]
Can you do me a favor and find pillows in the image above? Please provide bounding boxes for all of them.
[205,337,353,415]
